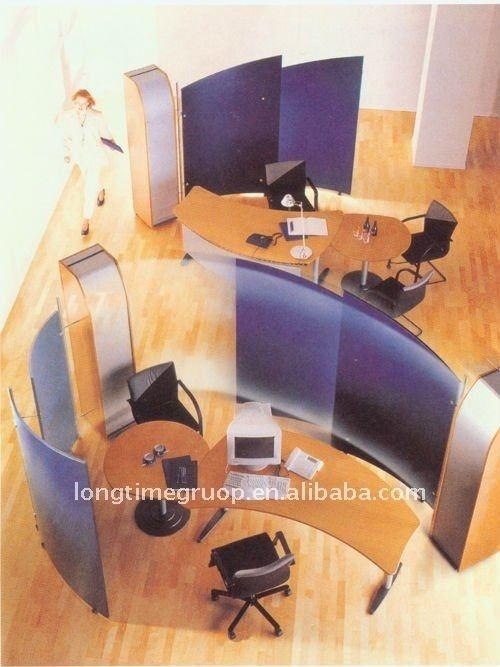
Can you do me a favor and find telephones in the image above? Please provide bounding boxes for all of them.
[246,233,273,249]
[283,446,324,481]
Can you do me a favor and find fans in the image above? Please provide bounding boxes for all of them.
[103,420,209,537]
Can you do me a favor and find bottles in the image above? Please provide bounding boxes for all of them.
[362,217,378,236]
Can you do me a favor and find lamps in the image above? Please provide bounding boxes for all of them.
[281,193,313,260]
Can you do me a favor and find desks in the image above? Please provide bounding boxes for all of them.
[173,186,344,285]
[178,430,431,615]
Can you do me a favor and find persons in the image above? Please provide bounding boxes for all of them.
[63,89,116,235]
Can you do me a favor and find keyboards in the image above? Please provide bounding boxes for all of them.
[222,471,291,501]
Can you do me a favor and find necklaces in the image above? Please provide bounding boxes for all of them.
[77,111,91,128]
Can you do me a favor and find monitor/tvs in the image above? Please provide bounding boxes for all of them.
[227,402,281,472]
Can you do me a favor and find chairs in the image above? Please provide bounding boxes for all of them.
[208,531,296,640]
[126,360,203,438]
[257,159,318,211]
[363,269,434,337]
[386,199,458,284]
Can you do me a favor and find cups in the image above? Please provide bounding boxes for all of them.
[143,443,165,465]
[352,223,369,244]
